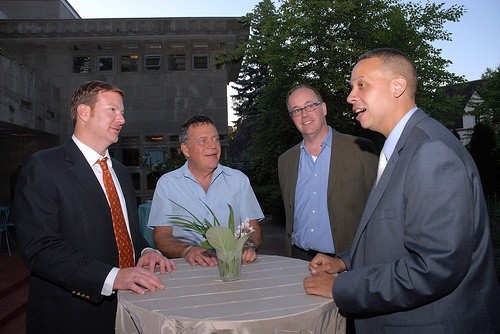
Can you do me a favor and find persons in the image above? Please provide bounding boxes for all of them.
[147,115,265,267]
[304,47,500,334]
[277,84,379,272]
[14,80,176,334]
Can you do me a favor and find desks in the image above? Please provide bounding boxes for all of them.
[115,254,347,334]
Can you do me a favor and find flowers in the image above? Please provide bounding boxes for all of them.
[165,198,256,281]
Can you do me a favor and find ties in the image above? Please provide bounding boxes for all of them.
[96,157,135,268]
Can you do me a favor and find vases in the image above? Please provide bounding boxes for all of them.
[215,251,242,281]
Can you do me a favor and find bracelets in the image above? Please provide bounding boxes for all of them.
[244,242,257,251]
[150,249,162,254]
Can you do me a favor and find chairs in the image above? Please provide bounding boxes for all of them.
[0,204,16,256]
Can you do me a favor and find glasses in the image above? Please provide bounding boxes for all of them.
[290,101,322,117]
